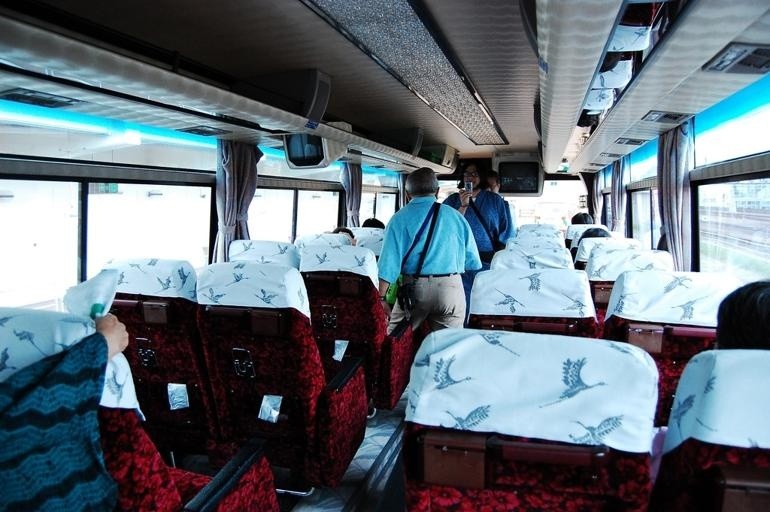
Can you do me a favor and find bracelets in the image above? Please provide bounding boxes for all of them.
[461,204,469,208]
[378,294,388,302]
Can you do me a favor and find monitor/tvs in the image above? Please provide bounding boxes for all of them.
[491,151,545,197]
[283,121,353,170]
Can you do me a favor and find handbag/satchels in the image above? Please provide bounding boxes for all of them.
[397,283,416,312]
[492,240,506,252]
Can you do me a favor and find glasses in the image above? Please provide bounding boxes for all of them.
[463,171,479,177]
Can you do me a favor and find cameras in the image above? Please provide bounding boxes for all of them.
[465,181,473,192]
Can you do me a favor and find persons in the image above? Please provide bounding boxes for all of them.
[486,169,516,241]
[571,212,594,224]
[577,229,611,245]
[333,227,357,247]
[0,312,133,511]
[441,161,508,328]
[361,218,385,228]
[371,168,480,348]
[715,280,769,350]
